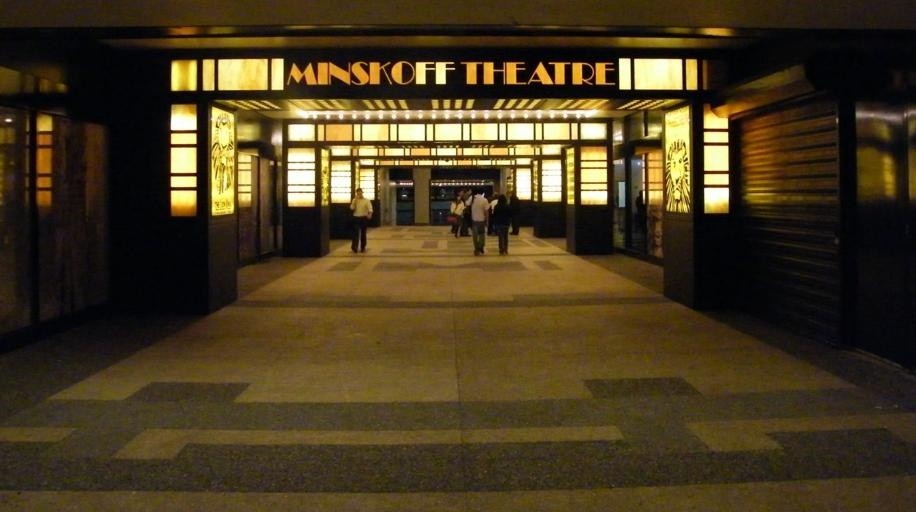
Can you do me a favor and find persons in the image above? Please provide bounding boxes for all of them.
[635,189,644,214]
[448,186,520,256]
[350,188,373,253]
